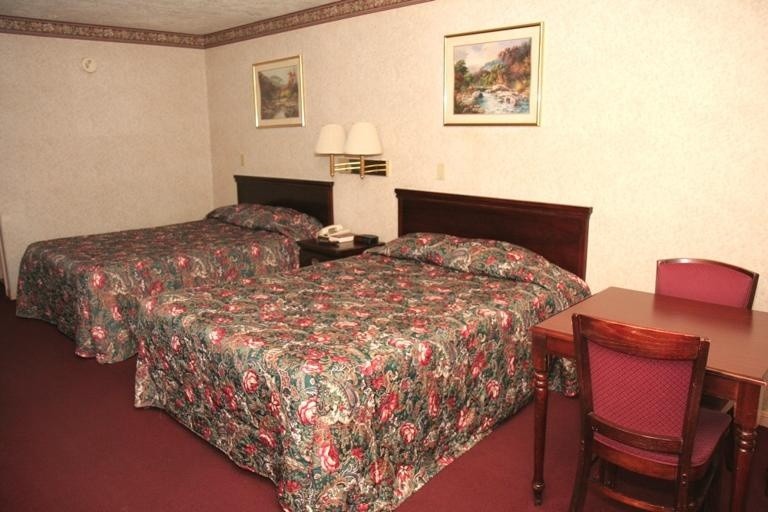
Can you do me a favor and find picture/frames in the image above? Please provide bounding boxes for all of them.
[442,22,544,126]
[253,54,304,128]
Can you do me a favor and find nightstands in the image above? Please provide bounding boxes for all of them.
[295,234,385,268]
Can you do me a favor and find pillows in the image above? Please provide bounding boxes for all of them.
[365,232,571,291]
[208,202,323,240]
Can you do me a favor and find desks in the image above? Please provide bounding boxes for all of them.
[531,286,767,512]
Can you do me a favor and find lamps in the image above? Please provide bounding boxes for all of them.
[315,123,388,180]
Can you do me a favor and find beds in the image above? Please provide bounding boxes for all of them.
[17,174,335,367]
[135,188,593,512]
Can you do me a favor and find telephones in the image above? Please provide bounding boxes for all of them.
[317,224,354,244]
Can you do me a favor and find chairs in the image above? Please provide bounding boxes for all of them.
[567,312,731,512]
[655,257,759,471]
